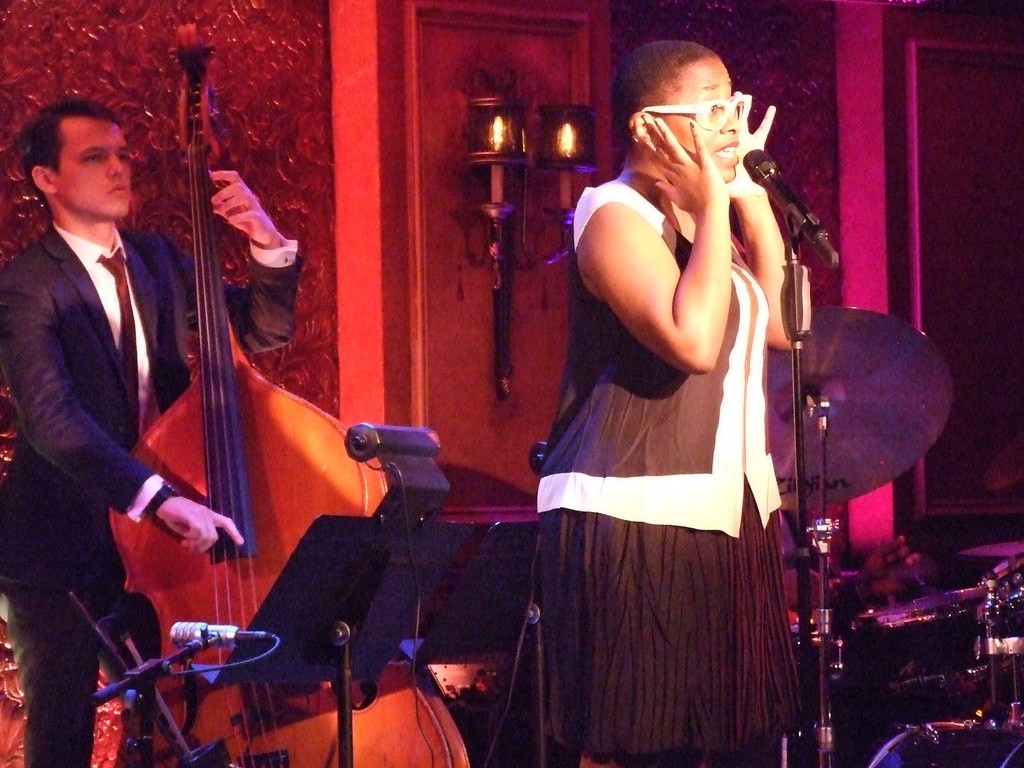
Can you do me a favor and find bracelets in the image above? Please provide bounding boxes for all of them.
[145,482,179,515]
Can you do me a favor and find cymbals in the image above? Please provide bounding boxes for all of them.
[761,305,954,509]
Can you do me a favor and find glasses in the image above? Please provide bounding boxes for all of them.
[643,90,745,131]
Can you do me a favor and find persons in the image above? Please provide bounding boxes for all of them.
[535,38,813,768]
[772,510,923,613]
[1,99,304,768]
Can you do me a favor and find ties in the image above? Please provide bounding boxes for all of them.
[97,247,139,445]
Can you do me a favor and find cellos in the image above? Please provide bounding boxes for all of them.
[106,29,470,768]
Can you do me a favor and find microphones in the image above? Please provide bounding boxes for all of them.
[742,149,839,269]
[170,621,276,651]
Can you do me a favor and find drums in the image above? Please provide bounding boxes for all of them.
[868,719,1024,768]
[844,579,1024,697]
